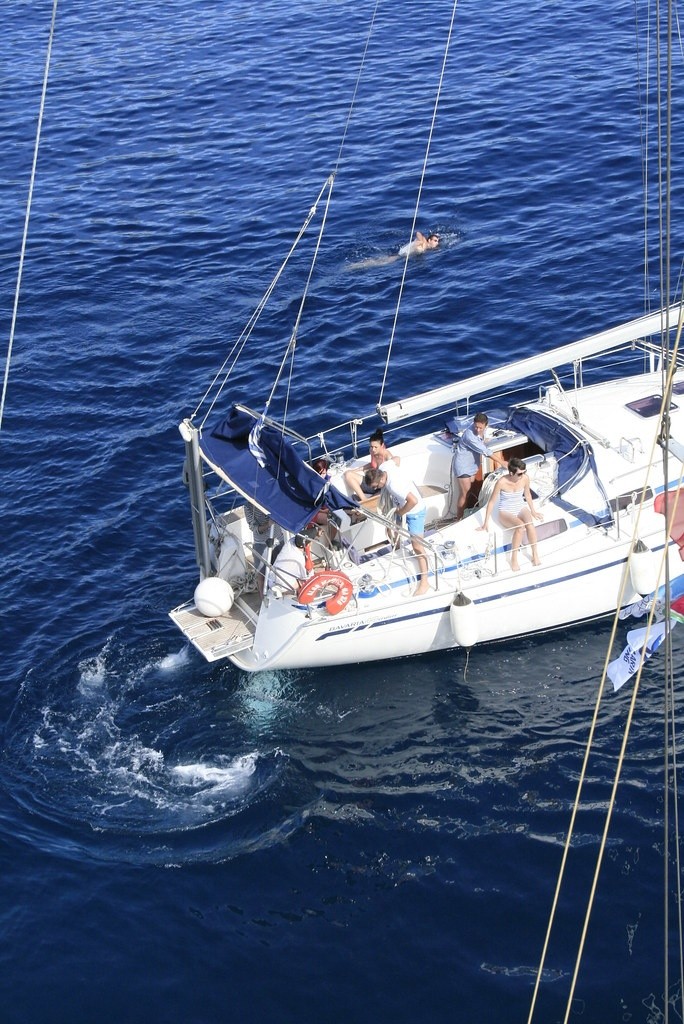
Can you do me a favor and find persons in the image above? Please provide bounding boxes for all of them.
[304,426,431,597]
[453,413,508,521]
[347,231,439,269]
[244,500,285,601]
[474,458,545,571]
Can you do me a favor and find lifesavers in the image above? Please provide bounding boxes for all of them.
[297,571,355,617]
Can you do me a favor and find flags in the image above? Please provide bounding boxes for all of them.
[604,486,684,693]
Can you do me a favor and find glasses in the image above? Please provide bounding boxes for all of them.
[372,483,379,490]
[514,470,527,477]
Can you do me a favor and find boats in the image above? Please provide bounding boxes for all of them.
[166,2,682,678]
